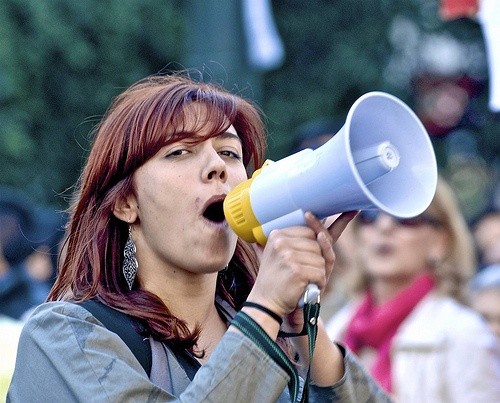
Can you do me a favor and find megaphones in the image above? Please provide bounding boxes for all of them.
[223,92,439,308]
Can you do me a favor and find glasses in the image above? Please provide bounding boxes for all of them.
[359,205,426,226]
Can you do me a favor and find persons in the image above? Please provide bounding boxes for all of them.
[3,74,395,402]
[326,170,499,403]
